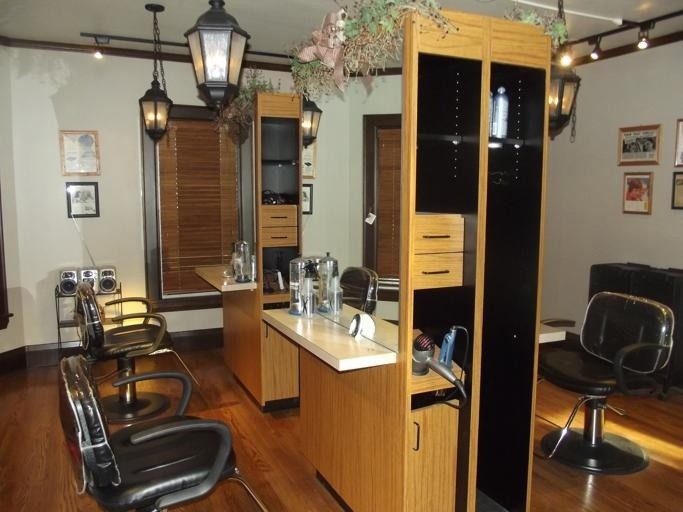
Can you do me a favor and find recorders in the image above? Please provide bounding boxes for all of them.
[58,266,117,296]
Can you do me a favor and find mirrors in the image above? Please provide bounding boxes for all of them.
[218,117,256,267]
[297,23,403,354]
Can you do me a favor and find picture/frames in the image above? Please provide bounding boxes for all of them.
[57,128,101,176]
[615,123,661,165]
[301,137,318,180]
[301,184,313,216]
[669,171,682,210]
[64,181,100,218]
[620,171,652,215]
[673,117,682,167]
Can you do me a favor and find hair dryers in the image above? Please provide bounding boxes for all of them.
[412,332,468,400]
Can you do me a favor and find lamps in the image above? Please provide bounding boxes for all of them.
[138,3,174,142]
[561,9,682,67]
[182,0,251,119]
[547,1,581,144]
[299,81,323,150]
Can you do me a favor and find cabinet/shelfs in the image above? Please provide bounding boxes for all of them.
[407,2,552,512]
[52,284,122,352]
[239,92,300,409]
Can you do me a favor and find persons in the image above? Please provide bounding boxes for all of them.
[629,137,656,152]
[626,182,643,200]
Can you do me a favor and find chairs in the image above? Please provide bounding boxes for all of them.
[72,282,171,427]
[338,264,378,314]
[54,355,272,511]
[536,289,676,476]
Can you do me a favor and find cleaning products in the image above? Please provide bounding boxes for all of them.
[299,262,320,318]
[328,259,345,315]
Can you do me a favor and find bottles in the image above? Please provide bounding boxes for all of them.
[488,83,509,138]
[230,241,256,284]
[287,251,345,318]
